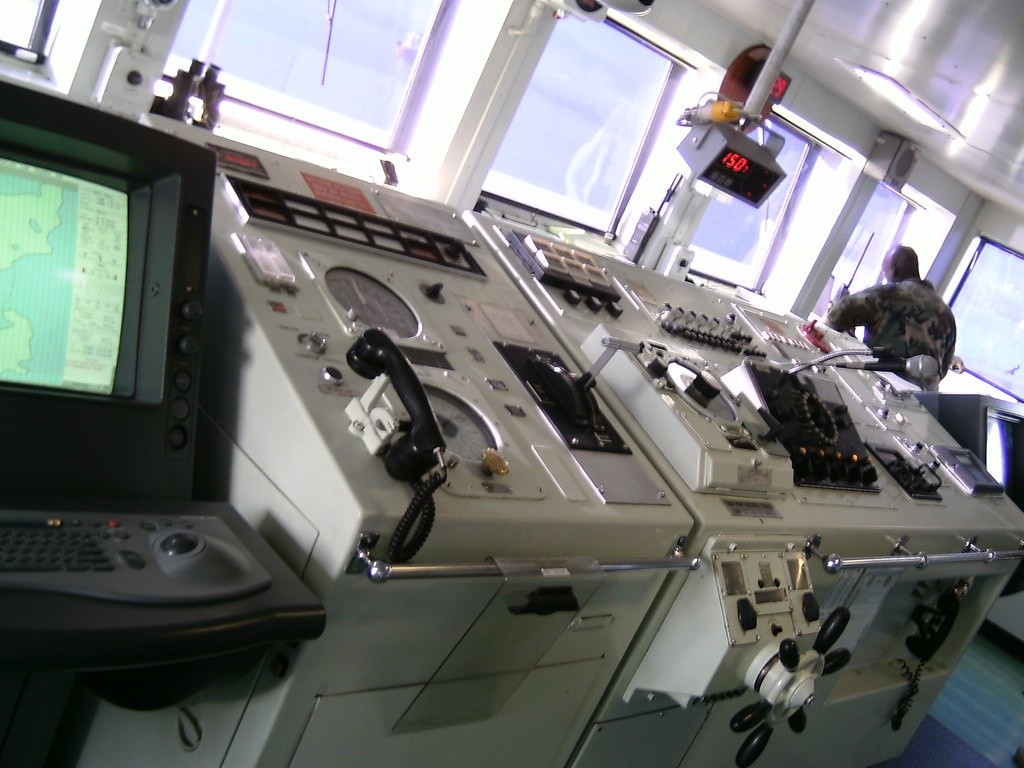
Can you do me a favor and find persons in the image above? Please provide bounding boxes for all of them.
[827,244,957,391]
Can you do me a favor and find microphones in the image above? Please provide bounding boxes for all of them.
[836,354,937,379]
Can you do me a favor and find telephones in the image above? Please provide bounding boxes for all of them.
[345,326,447,482]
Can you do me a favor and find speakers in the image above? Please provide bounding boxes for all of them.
[862,132,921,192]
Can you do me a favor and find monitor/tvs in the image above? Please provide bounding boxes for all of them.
[0,146,154,402]
[986,410,1024,512]
